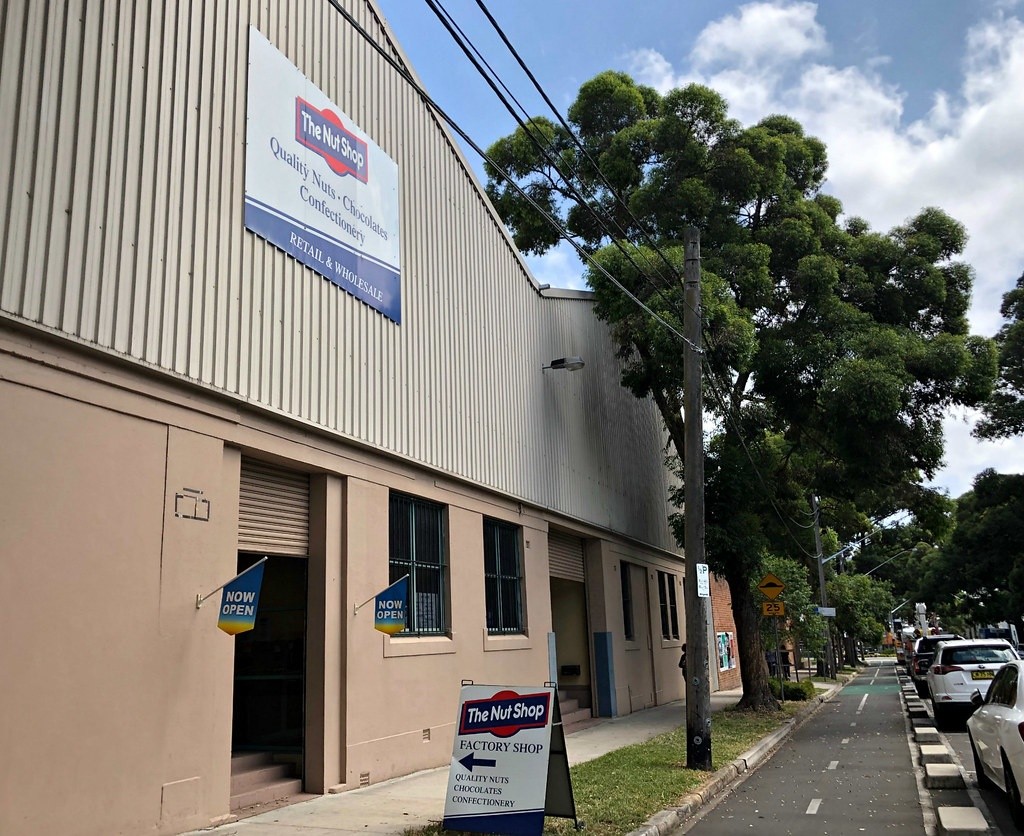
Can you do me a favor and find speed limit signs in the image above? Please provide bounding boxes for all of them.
[762,602,785,616]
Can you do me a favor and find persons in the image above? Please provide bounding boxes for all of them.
[678,643,686,682]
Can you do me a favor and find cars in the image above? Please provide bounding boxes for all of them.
[895,622,1024,724]
[966,660,1024,833]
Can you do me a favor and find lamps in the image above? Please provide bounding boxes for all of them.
[543,355,585,372]
